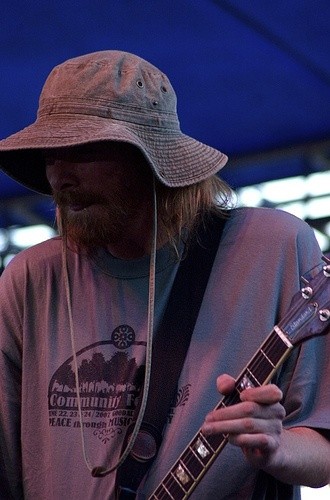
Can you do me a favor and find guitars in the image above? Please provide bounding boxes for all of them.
[147,253,330,500]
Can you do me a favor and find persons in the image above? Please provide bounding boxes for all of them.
[0,50,330,500]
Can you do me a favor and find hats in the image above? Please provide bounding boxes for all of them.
[1,49,229,198]
